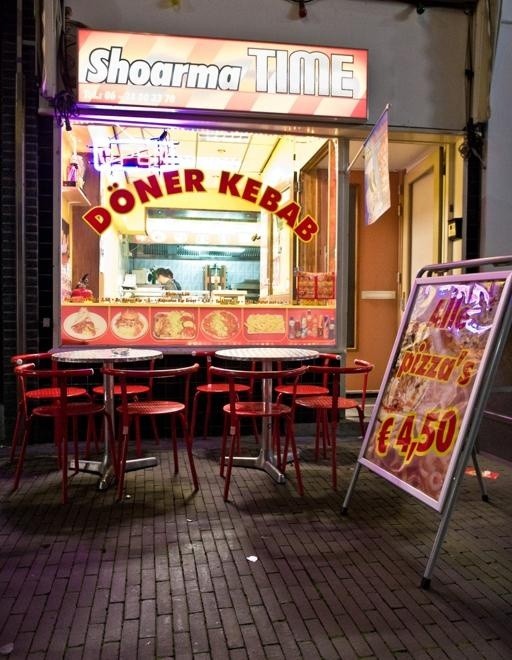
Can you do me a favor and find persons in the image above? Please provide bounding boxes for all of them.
[165,269,181,290]
[156,268,177,290]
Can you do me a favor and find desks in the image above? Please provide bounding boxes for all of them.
[215,348,320,484]
[52,348,164,493]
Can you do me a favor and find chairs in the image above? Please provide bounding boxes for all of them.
[14,364,114,500]
[188,351,260,458]
[208,366,304,501]
[11,353,94,472]
[282,357,375,492]
[99,363,200,501]
[273,352,341,463]
[91,358,159,459]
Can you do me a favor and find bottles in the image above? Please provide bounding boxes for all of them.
[288,310,339,339]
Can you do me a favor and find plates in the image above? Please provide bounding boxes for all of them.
[151,311,195,340]
[200,309,239,340]
[61,311,108,342]
[110,310,147,342]
